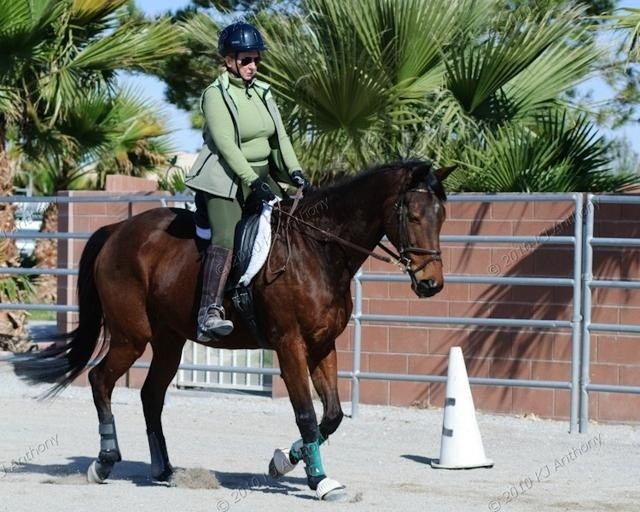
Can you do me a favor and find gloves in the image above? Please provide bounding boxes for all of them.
[291,170,309,191]
[248,176,275,203]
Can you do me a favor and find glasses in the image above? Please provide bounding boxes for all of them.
[231,54,262,66]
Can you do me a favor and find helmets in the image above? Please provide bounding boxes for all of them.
[218,21,268,56]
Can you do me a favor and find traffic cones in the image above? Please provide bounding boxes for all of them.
[427,342,497,472]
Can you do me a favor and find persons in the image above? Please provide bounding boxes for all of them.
[181,21,311,342]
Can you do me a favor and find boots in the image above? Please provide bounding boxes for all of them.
[196,243,236,344]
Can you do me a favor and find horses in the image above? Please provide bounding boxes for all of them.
[7,158,460,502]
